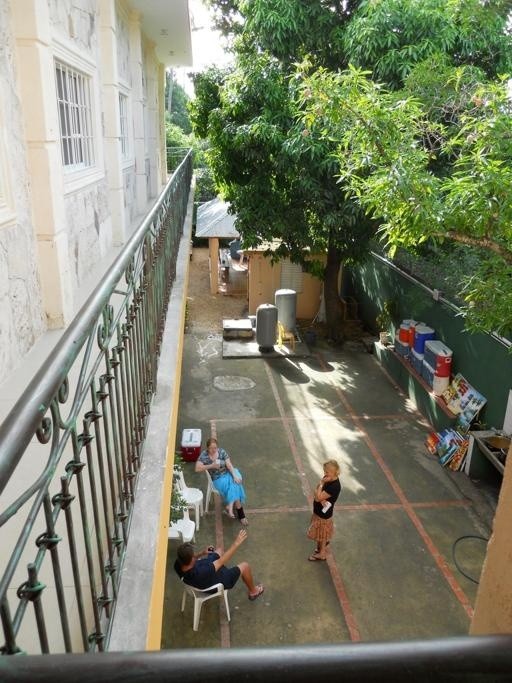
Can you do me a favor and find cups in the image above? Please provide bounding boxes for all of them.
[216,459,220,470]
[208,544,216,551]
[323,502,331,513]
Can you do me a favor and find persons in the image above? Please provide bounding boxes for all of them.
[307,459,341,560]
[194,438,249,525]
[174,529,265,602]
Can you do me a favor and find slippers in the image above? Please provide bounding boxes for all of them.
[222,508,236,519]
[240,517,249,526]
[307,554,327,562]
[248,583,264,600]
[326,540,330,546]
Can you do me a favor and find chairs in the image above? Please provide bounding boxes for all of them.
[168,506,196,545]
[178,577,231,631]
[205,468,242,513]
[173,464,204,531]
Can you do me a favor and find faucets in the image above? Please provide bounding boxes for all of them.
[491,427,502,436]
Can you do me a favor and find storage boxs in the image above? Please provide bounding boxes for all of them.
[180,428,202,462]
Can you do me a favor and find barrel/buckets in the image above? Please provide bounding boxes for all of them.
[436,353,452,377]
[433,375,449,396]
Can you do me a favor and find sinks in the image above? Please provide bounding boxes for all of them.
[478,436,511,474]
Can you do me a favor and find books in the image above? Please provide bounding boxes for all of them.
[444,373,487,423]
[425,428,468,471]
